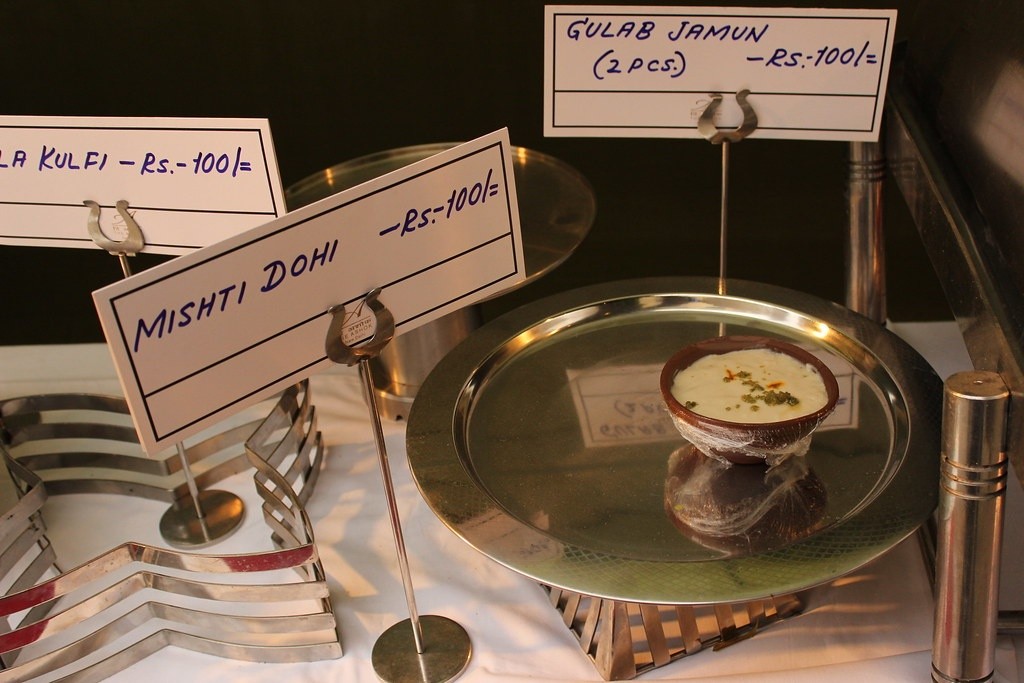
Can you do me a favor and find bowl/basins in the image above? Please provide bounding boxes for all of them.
[659,335,840,464]
[664,443,828,555]
